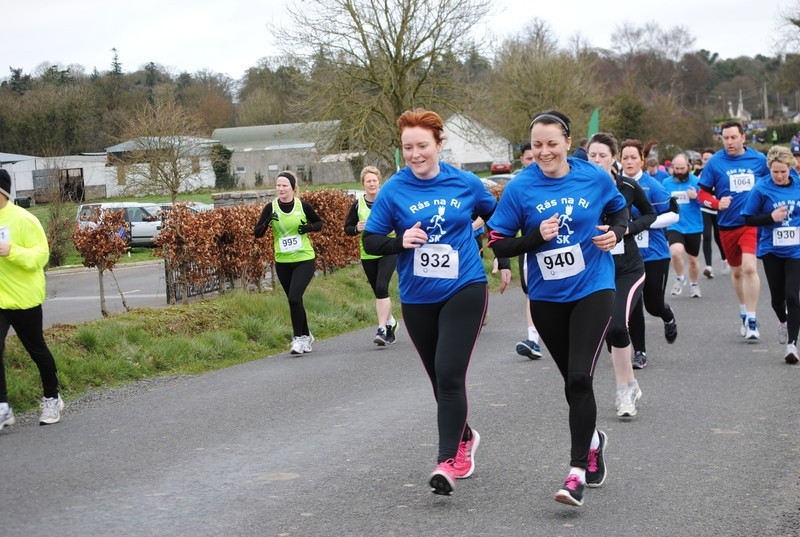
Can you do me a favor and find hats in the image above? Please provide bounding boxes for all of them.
[0,168,12,199]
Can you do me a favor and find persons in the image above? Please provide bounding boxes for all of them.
[362,108,512,496]
[567,118,800,278]
[697,121,771,340]
[514,142,544,361]
[486,111,628,508]
[619,139,677,368]
[254,170,323,355]
[588,131,657,416]
[344,166,400,348]
[742,144,800,365]
[0,169,64,427]
[662,151,702,298]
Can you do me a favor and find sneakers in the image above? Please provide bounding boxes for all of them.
[585,429,607,487]
[386,321,399,344]
[515,339,542,360]
[777,321,787,344]
[739,313,747,336]
[39,393,65,425]
[631,351,647,369]
[427,458,456,496]
[0,402,15,429]
[671,276,686,295]
[301,331,314,352]
[554,474,585,506]
[290,336,303,354]
[373,327,386,346]
[785,340,799,363]
[614,379,643,417]
[690,286,701,297]
[664,308,677,344]
[744,317,760,339]
[454,429,480,479]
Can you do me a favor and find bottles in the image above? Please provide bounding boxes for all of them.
[0,227,7,241]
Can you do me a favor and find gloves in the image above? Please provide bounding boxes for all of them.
[265,212,279,225]
[297,219,309,234]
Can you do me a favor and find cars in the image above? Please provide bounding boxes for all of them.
[490,160,515,175]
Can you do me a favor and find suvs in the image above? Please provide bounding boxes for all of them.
[73,201,215,247]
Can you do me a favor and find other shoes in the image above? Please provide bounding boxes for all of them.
[721,258,729,275]
[702,265,714,279]
